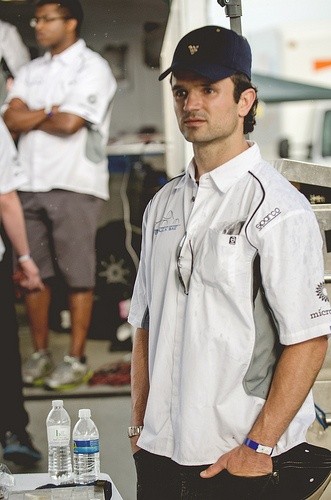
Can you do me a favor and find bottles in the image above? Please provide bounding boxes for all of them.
[72,409,99,484]
[45,399,72,480]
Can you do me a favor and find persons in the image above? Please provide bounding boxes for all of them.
[0,116,45,467]
[128,25,331,500]
[1,0,118,393]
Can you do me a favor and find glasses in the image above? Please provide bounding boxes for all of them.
[30,17,69,26]
[176,233,193,294]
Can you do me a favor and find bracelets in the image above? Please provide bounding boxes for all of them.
[18,252,31,262]
[128,426,144,439]
[244,437,273,455]
[44,104,52,115]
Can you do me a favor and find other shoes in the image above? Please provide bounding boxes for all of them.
[21,353,53,381]
[45,358,88,387]
[5,433,42,465]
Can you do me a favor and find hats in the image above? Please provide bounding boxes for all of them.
[159,26,251,80]
